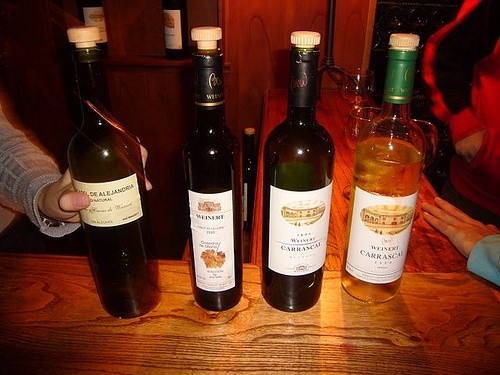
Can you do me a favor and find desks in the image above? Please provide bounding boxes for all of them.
[0,85,500,375]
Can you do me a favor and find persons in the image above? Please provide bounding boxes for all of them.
[1,104,154,239]
[420,195,500,288]
[421,1,500,232]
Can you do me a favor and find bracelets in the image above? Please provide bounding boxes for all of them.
[38,211,70,228]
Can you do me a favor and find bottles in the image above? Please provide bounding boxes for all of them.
[340,34,425,303]
[261,31,335,314]
[79,0,108,57]
[162,0,189,59]
[66,27,162,318]
[239,128,257,231]
[182,26,243,311]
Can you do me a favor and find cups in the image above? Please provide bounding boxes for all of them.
[343,69,375,107]
[345,106,382,150]
[410,120,437,171]
